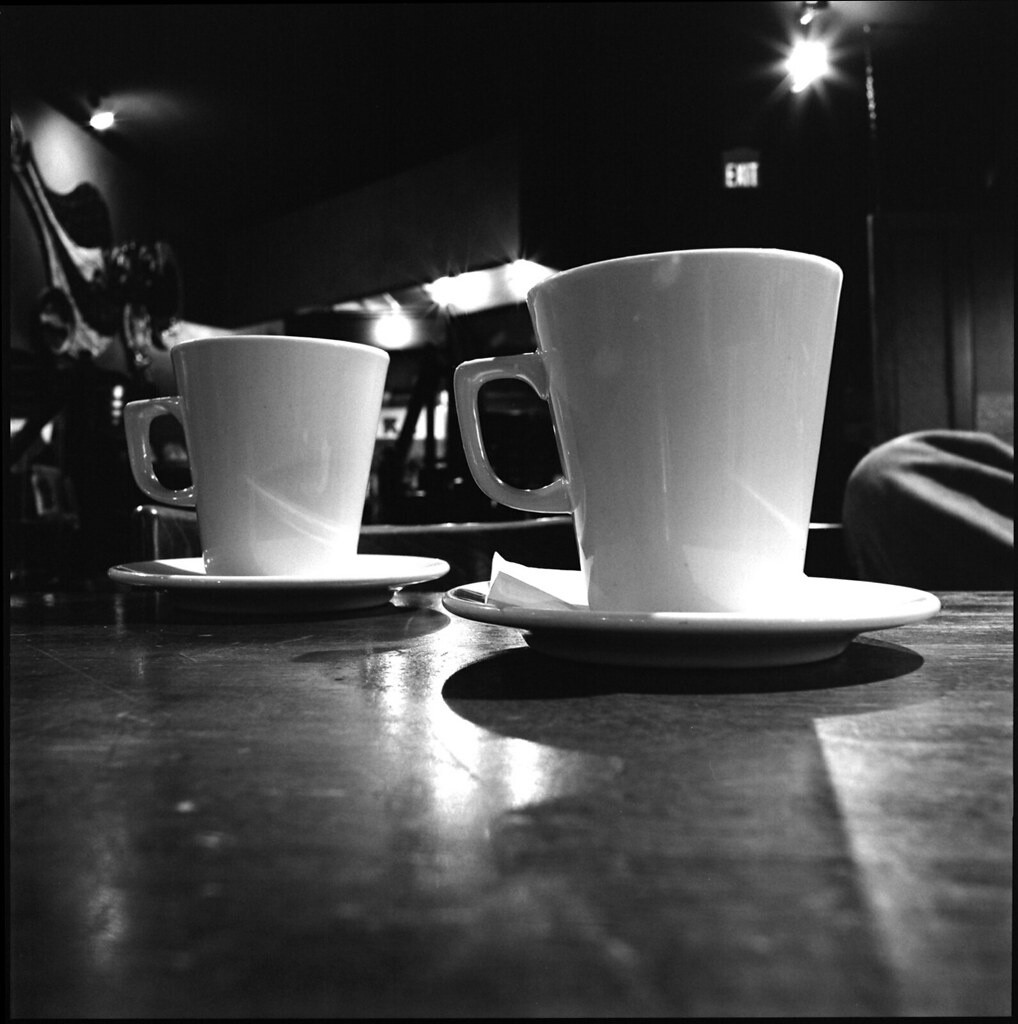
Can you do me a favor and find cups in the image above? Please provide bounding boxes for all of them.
[452,248,843,616]
[123,334,390,575]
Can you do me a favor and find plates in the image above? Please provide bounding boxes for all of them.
[107,552,450,610]
[436,575,942,667]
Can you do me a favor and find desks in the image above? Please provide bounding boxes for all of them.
[8,588,1014,1015]
[133,505,575,592]
